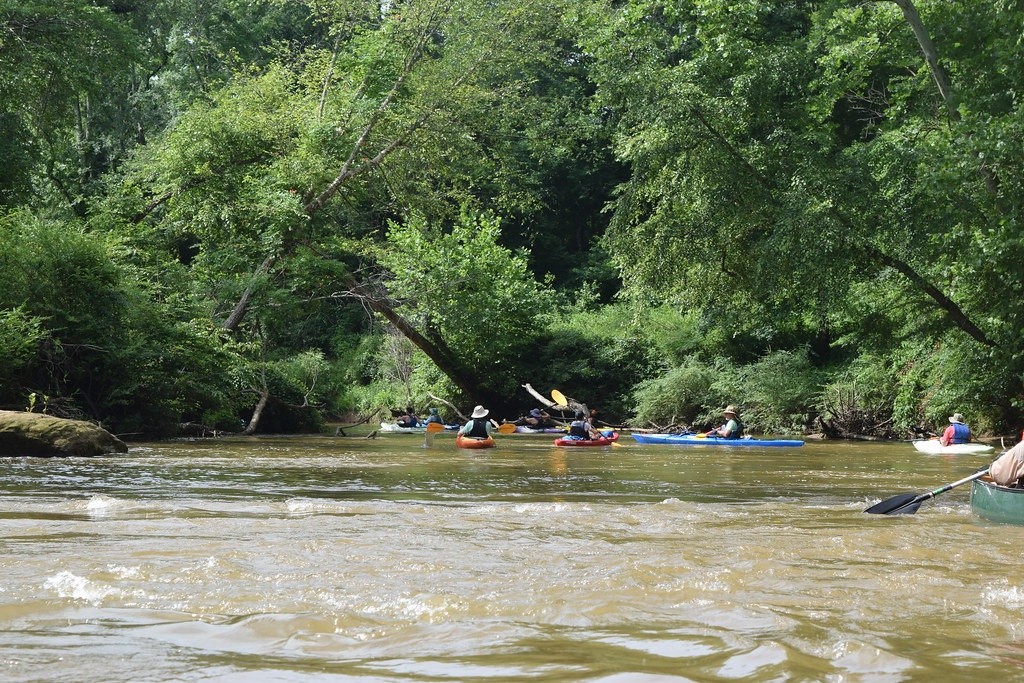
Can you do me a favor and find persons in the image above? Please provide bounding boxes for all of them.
[524,405,551,430]
[987,429,1024,489]
[939,412,971,446]
[570,412,597,440]
[457,405,493,439]
[425,408,444,425]
[708,403,742,441]
[588,408,599,428]
[397,406,422,428]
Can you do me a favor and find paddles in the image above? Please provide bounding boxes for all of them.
[697,426,722,438]
[862,468,990,514]
[550,389,620,448]
[903,435,1017,442]
[427,421,518,434]
[514,403,558,424]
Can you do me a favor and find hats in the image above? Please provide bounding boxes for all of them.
[724,405,737,414]
[471,405,489,418]
[429,408,438,415]
[575,411,586,417]
[407,407,413,414]
[949,413,965,424]
[530,408,542,417]
[591,410,599,415]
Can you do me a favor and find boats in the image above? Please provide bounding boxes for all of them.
[631,430,806,447]
[379,421,426,434]
[416,419,461,431]
[456,434,497,450]
[969,464,1024,526]
[516,425,570,435]
[910,439,996,456]
[554,431,621,448]
